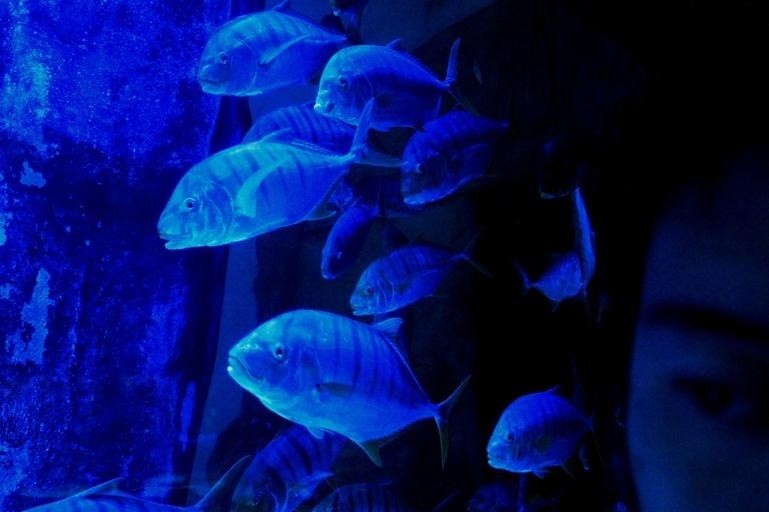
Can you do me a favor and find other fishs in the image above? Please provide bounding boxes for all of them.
[155,0,495,512]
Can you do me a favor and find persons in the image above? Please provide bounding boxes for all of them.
[610,115,769,509]
